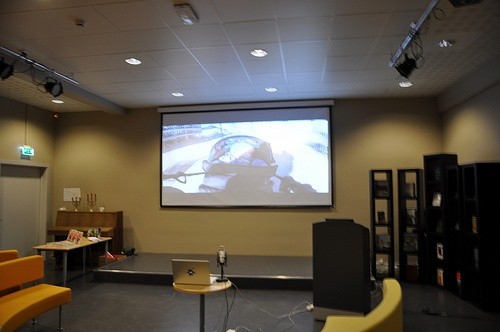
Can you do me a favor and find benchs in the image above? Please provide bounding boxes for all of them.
[0,255,72,332]
[0,250,23,290]
[320,278,403,332]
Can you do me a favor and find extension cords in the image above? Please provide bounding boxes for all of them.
[306,304,313,311]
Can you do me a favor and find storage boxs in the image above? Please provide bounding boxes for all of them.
[99,255,127,267]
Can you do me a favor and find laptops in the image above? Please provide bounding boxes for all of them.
[171,259,218,286]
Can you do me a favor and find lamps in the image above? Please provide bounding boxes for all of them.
[87,193,96,212]
[72,197,81,212]
[37,75,63,97]
[394,58,417,79]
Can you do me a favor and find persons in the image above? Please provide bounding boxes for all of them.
[161,135,316,194]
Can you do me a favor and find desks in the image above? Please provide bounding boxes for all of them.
[173,276,232,332]
[32,237,112,286]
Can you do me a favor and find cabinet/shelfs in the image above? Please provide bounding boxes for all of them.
[397,154,500,312]
[370,170,395,281]
[47,210,123,254]
